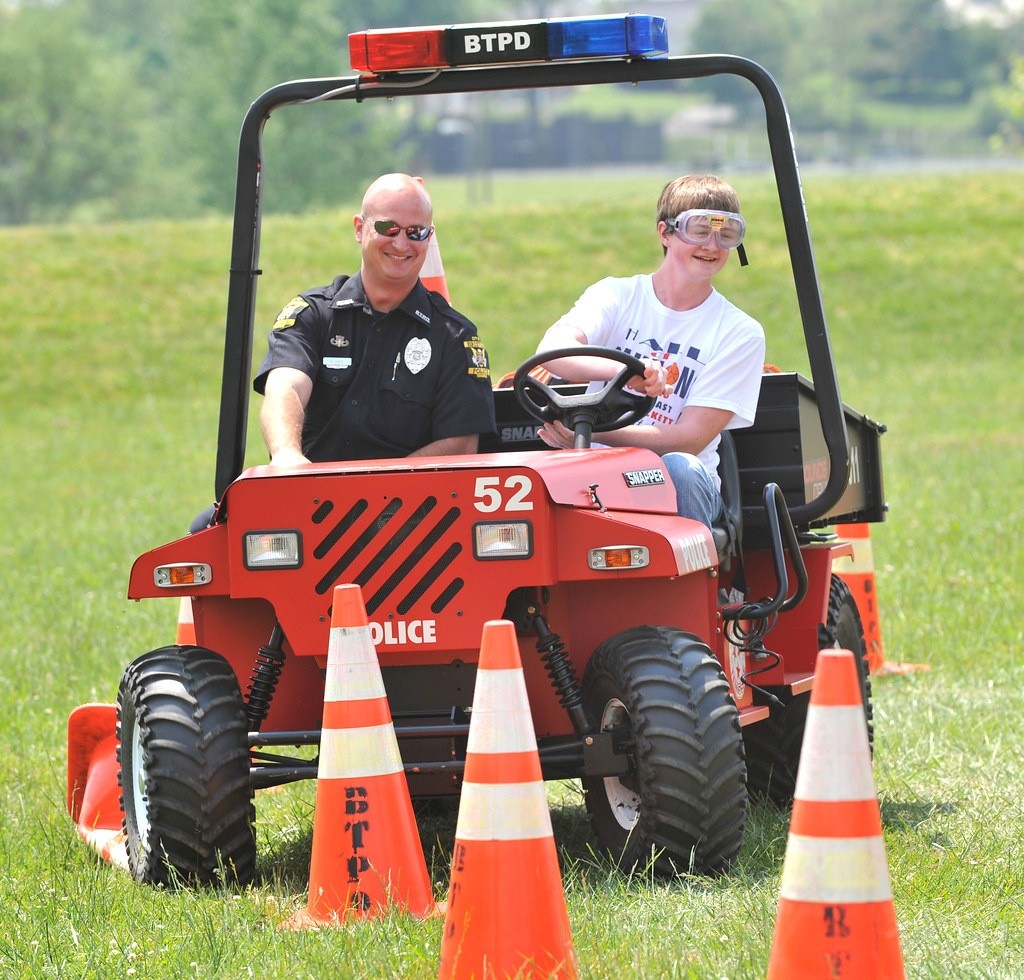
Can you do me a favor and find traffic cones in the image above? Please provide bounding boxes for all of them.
[307,584,435,932]
[416,174,448,304]
[177,590,199,648]
[58,704,129,872]
[765,646,907,980]
[435,617,578,980]
[828,521,922,674]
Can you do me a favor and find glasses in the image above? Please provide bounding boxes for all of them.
[661,209,749,267]
[360,214,433,241]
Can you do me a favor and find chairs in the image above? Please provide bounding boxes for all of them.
[711,432,744,560]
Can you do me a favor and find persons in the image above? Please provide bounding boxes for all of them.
[189,172,501,532]
[533,173,766,536]
